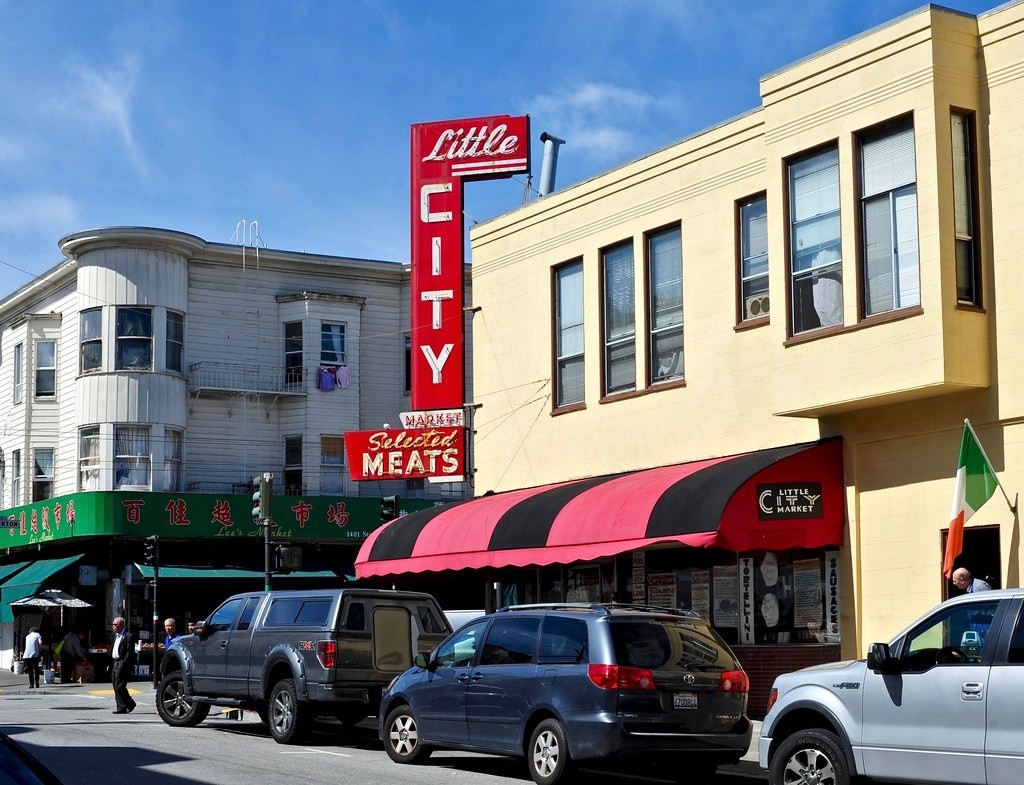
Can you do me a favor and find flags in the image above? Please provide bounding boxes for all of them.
[944,422,997,579]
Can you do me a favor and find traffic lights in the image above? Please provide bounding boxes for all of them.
[252,472,272,519]
[144,535,159,565]
[381,495,399,522]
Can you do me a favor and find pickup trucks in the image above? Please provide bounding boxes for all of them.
[155,587,456,745]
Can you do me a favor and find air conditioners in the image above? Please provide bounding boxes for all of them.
[744,291,769,319]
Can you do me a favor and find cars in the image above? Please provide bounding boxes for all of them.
[757,588,1024,785]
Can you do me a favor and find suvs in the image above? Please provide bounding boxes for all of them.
[378,603,753,785]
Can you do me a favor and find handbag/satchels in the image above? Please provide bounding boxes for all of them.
[54,631,71,655]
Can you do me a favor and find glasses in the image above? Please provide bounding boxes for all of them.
[111,624,121,627]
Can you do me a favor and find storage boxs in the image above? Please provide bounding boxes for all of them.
[134,664,150,676]
[75,665,94,682]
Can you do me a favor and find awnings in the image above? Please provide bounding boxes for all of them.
[354,436,845,579]
[0,561,32,580]
[0,553,85,623]
[126,544,338,579]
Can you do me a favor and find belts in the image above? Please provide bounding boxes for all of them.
[114,659,120,661]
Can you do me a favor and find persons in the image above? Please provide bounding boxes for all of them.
[60,631,87,683]
[23,627,42,688]
[952,568,996,656]
[110,617,136,714]
[164,618,180,651]
[187,617,196,634]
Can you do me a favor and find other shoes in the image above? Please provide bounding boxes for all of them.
[113,707,127,714]
[126,702,136,713]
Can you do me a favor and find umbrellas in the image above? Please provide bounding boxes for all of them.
[6,589,93,627]
